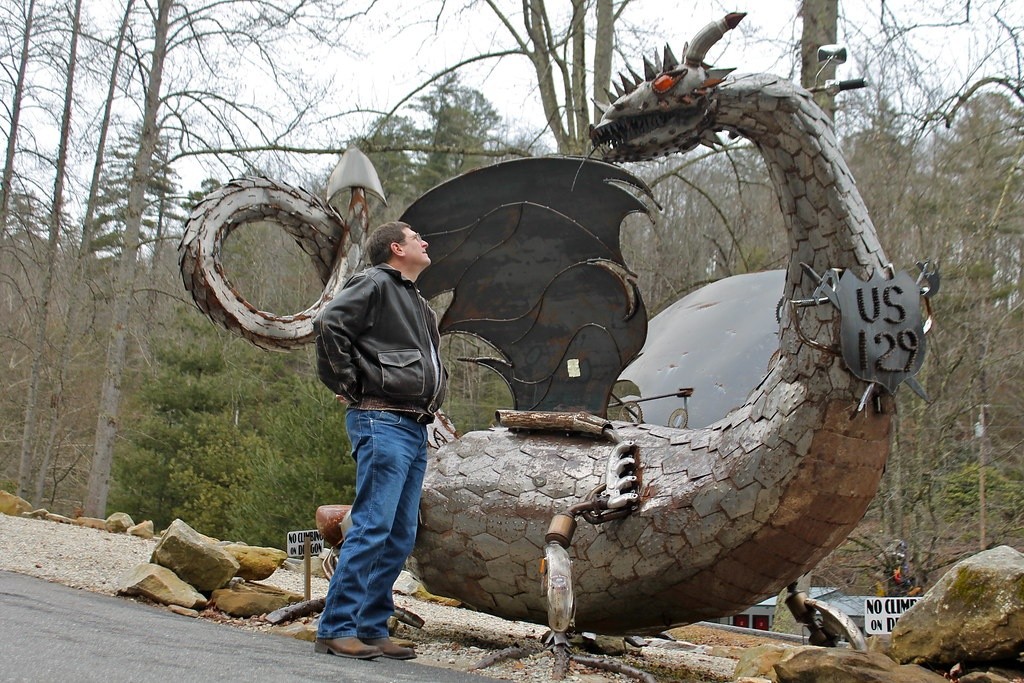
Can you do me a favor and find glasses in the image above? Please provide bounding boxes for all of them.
[395,232,421,244]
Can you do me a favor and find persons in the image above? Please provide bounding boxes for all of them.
[314,221,449,659]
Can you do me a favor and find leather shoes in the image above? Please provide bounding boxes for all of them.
[361,635,417,660]
[314,635,384,659]
